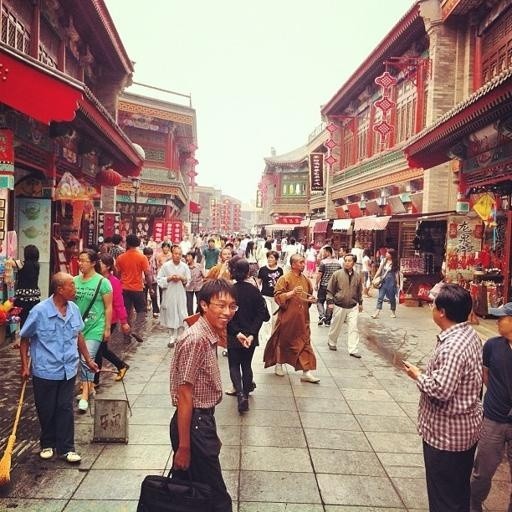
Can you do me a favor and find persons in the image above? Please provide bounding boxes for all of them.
[18,270,99,463]
[400,282,481,512]
[168,276,241,511]
[468,299,512,511]
[64,230,398,412]
[11,241,42,329]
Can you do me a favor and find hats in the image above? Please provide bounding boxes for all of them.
[491,302,512,317]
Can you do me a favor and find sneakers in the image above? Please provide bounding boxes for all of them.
[124,335,131,344]
[328,343,336,350]
[168,342,174,348]
[40,448,53,459]
[226,383,256,410]
[132,331,143,342]
[275,366,284,376]
[301,374,321,382]
[351,352,361,358]
[115,364,129,382]
[77,383,99,411]
[318,313,330,327]
[64,451,82,463]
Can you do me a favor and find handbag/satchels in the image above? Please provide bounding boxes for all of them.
[373,276,383,288]
[137,475,212,512]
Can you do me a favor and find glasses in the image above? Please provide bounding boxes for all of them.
[211,303,238,312]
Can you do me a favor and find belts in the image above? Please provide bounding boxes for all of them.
[194,408,215,415]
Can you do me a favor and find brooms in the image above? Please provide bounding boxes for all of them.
[0,358,31,483]
